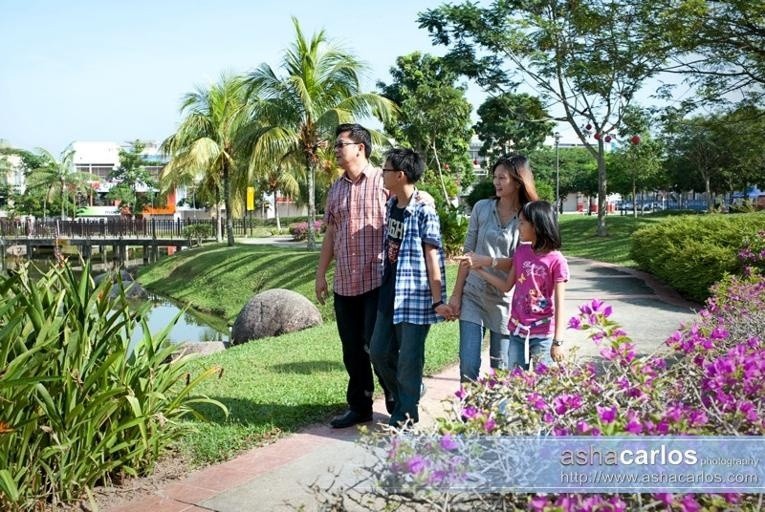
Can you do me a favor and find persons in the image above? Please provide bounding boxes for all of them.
[25,212,34,239]
[368,148,454,428]
[447,151,541,382]
[314,123,437,428]
[474,200,570,370]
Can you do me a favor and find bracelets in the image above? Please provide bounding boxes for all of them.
[432,300,443,309]
[491,257,498,269]
[553,339,564,346]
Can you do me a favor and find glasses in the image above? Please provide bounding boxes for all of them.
[334,142,360,148]
[383,168,400,173]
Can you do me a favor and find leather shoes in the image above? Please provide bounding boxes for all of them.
[386,392,395,414]
[330,410,372,427]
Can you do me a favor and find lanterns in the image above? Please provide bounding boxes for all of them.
[605,136,611,142]
[594,133,598,139]
[586,125,591,129]
[632,135,640,144]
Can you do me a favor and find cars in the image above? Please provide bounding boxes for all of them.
[614,199,662,210]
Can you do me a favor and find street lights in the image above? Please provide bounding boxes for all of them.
[555,132,562,221]
